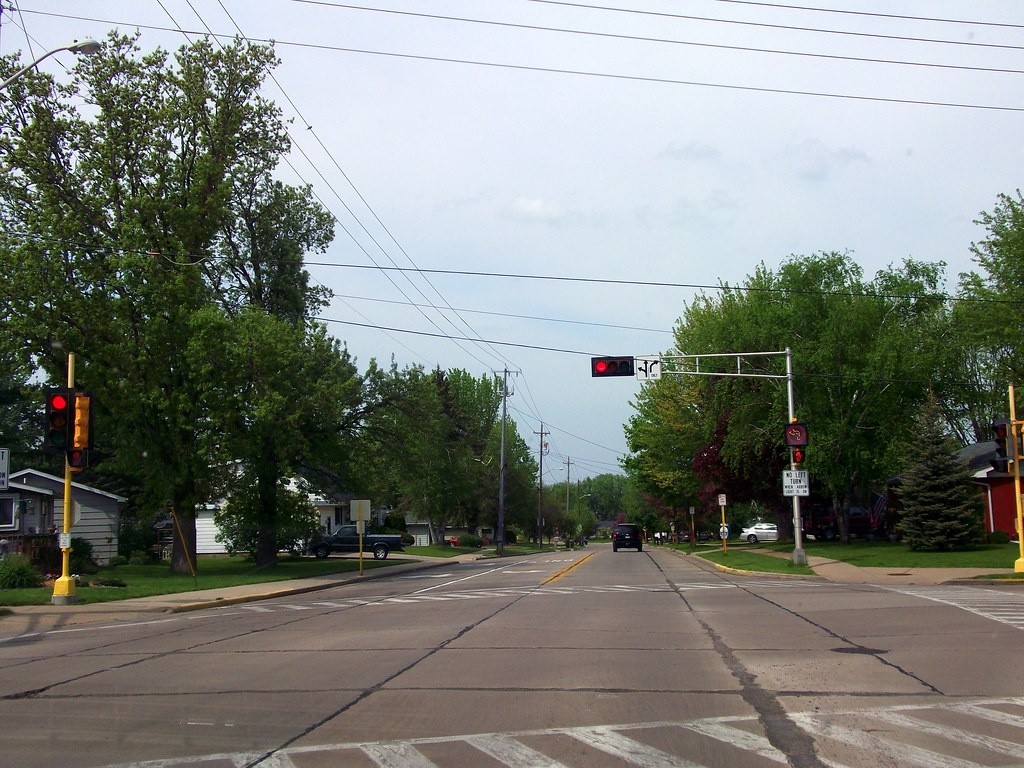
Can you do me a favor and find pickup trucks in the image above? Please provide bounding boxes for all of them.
[308,525,405,560]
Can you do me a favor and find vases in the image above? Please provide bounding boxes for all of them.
[48,528,57,533]
[105,536,114,543]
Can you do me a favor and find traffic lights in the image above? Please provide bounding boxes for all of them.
[48,391,71,449]
[792,449,806,463]
[590,355,635,378]
[988,419,1010,474]
[65,449,87,467]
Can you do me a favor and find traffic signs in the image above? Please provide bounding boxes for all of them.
[635,354,662,380]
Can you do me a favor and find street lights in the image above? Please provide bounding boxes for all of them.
[470,458,506,553]
[577,493,592,545]
[539,467,564,549]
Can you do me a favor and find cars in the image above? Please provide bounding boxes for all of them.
[739,524,805,544]
[446,534,489,548]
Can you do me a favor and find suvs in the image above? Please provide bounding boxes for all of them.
[610,523,644,552]
